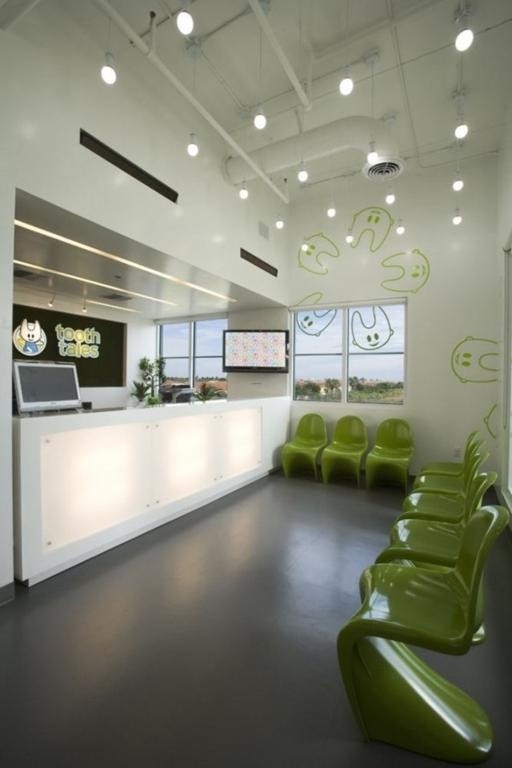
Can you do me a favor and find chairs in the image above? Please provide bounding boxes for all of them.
[375,471,497,568]
[418,431,480,475]
[337,505,510,763]
[321,416,368,488]
[411,439,487,494]
[396,451,491,523]
[365,418,414,497]
[281,413,328,481]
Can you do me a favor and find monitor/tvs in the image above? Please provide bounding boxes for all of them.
[222,329,288,374]
[13,362,80,412]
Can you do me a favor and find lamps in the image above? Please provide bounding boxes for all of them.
[82,302,88,313]
[48,296,55,307]
[0,0,477,253]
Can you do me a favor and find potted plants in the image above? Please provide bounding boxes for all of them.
[139,357,167,408]
[130,381,151,408]
